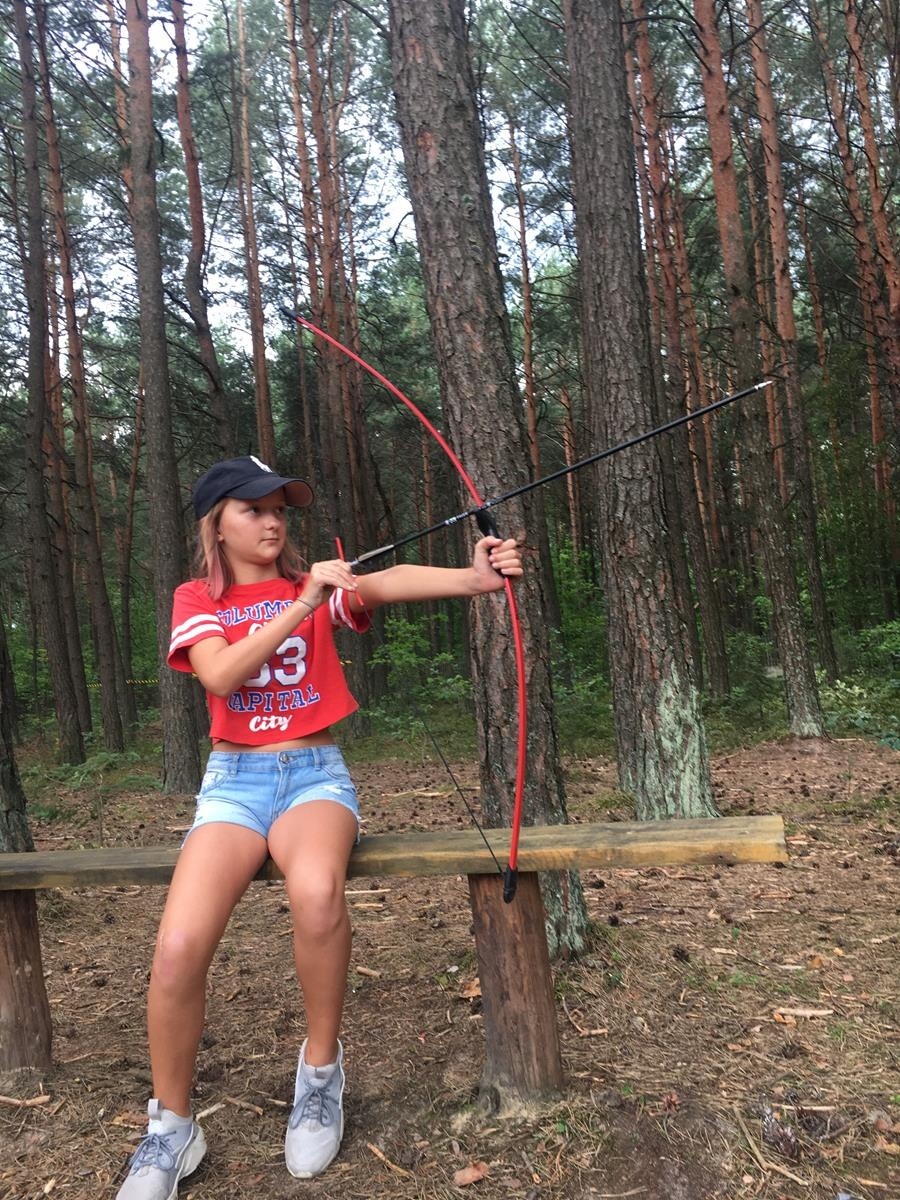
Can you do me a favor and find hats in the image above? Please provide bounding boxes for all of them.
[192,454,315,521]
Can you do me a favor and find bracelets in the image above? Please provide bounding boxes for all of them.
[296,597,316,611]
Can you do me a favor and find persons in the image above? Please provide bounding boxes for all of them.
[116,453,524,1200]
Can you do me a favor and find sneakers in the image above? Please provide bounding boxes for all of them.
[116,1098,208,1200]
[284,1035,346,1179]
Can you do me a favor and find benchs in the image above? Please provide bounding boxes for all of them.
[0,815,790,1118]
